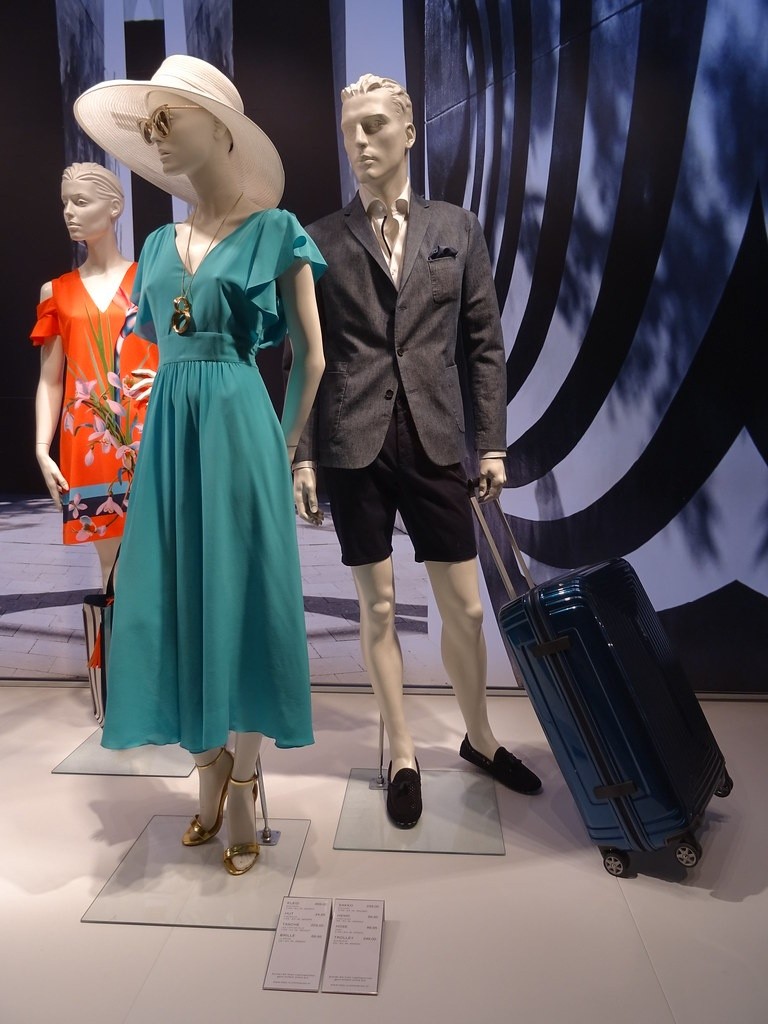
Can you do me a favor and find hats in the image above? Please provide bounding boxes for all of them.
[74,54,286,208]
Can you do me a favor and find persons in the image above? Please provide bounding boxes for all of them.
[32,54,326,875]
[291,74,544,830]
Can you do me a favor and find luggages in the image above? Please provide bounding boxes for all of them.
[470,493,734,875]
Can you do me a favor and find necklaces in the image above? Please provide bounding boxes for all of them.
[172,192,243,333]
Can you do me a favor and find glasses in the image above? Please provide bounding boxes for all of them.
[136,103,204,146]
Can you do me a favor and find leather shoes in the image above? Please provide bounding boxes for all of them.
[458,733,543,793]
[387,756,423,829]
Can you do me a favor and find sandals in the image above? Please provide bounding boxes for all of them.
[181,747,235,846]
[222,773,259,876]
